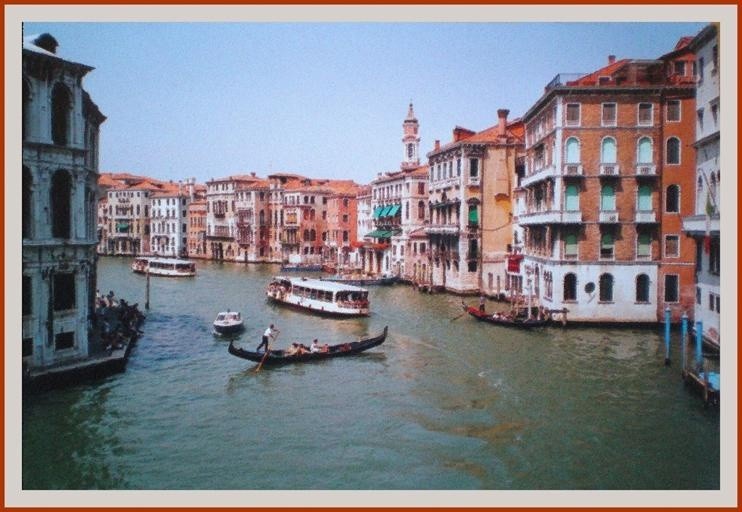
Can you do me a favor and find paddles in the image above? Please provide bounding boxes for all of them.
[450,312,468,323]
[256,330,281,371]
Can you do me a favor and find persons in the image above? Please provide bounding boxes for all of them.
[479,292,485,312]
[256,323,278,352]
[95,289,146,349]
[286,338,328,356]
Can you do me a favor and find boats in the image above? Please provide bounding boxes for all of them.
[228,325,388,364]
[130,257,197,277]
[461,303,547,328]
[267,263,401,318]
[212,311,244,337]
